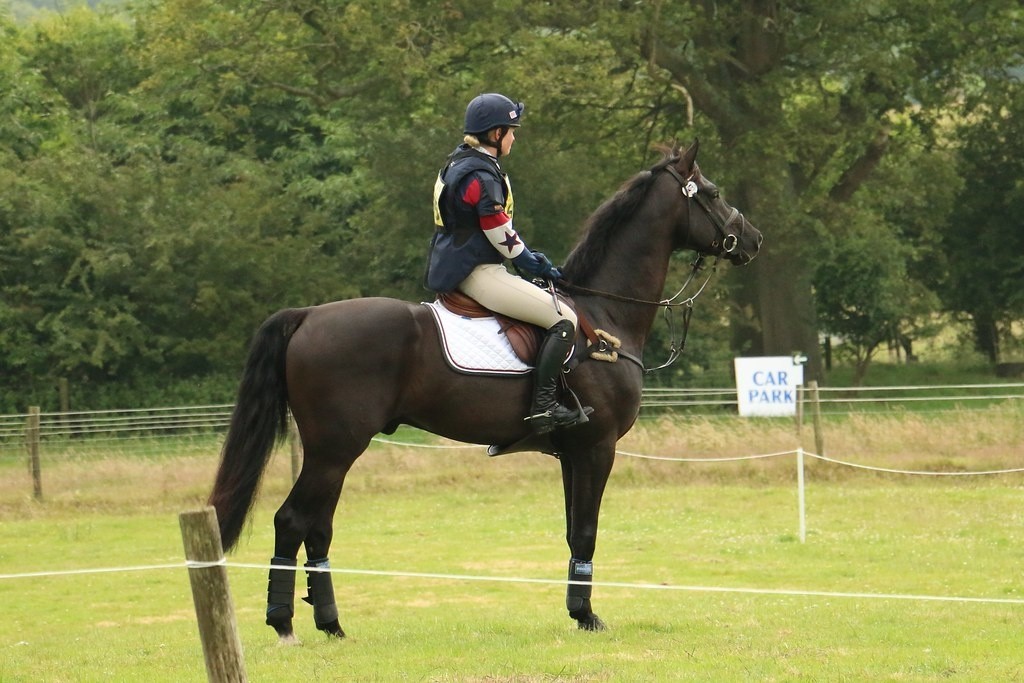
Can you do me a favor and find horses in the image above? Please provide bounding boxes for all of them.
[207,139,764,648]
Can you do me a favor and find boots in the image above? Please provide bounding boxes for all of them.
[529,318,595,436]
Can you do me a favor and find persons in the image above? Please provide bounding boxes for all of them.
[423,92,592,427]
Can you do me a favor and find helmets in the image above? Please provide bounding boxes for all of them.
[463,92,524,134]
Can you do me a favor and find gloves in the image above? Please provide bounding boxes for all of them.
[511,246,563,285]
